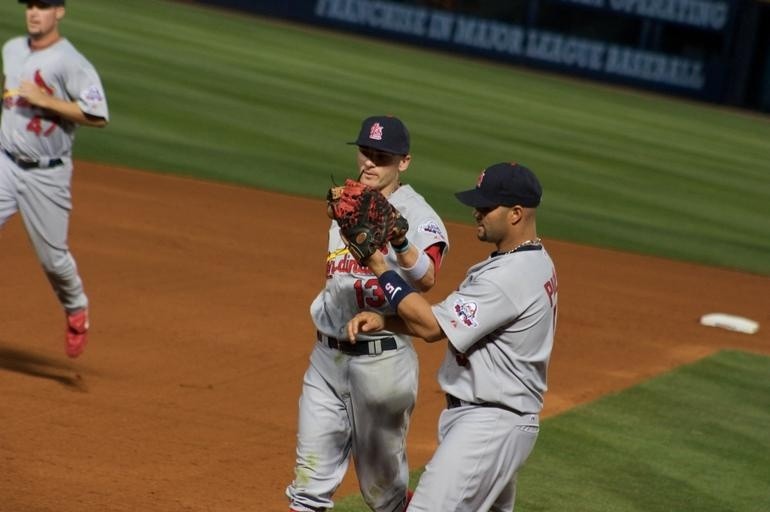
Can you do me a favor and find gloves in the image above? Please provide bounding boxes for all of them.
[339,224,379,268]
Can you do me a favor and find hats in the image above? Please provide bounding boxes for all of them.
[346,111,411,156]
[454,162,544,209]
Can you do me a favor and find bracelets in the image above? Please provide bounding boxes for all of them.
[378,269,417,316]
[391,237,409,256]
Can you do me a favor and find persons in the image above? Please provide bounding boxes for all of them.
[287,114,450,512]
[346,162,558,512]
[1,0,110,360]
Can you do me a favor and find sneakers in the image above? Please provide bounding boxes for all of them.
[63,305,91,358]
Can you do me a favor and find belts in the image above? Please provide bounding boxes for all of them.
[445,393,524,417]
[316,328,397,355]
[4,148,64,170]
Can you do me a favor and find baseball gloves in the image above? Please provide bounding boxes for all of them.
[325,176,394,265]
